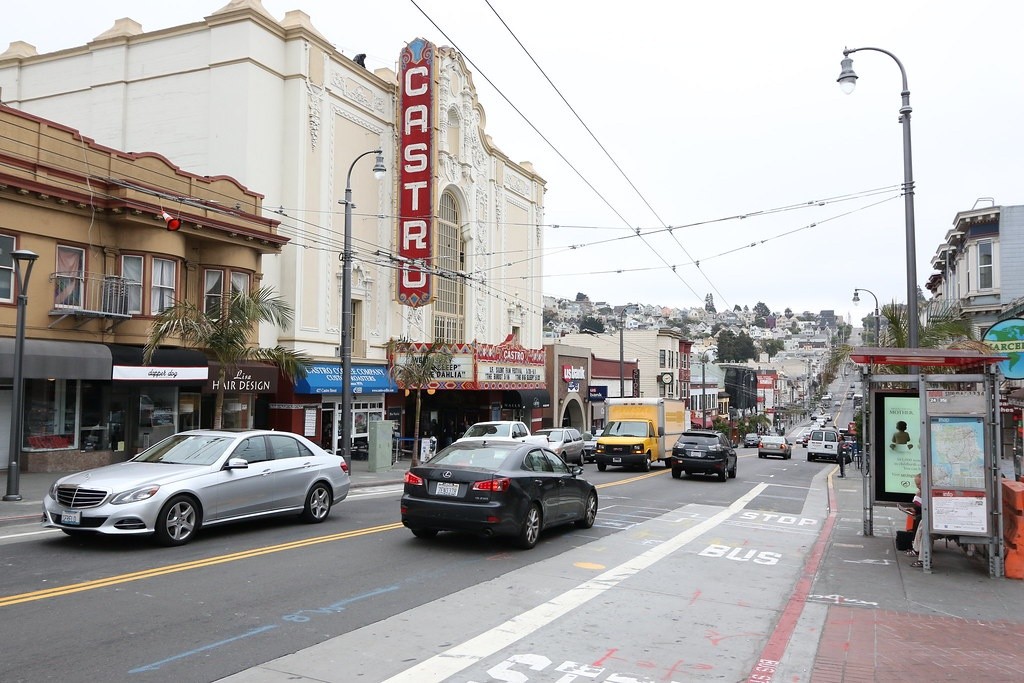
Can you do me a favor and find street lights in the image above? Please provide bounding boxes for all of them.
[854,287,878,346]
[620,304,637,398]
[838,46,921,372]
[702,348,719,430]
[339,150,386,466]
[1,250,40,501]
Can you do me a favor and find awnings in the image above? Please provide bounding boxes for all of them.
[179,361,278,393]
[0,336,112,379]
[294,365,398,393]
[107,342,209,379]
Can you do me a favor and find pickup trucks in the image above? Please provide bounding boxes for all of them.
[452,421,549,448]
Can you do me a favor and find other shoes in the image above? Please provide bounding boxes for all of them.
[912,561,933,568]
[904,548,919,557]
[838,476,845,480]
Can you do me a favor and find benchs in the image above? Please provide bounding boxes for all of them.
[961,537,998,545]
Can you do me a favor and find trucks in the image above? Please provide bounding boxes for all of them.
[595,398,685,472]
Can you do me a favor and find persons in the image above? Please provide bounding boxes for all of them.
[905,473,955,567]
[430,419,438,436]
[837,434,845,477]
[458,421,469,438]
[444,418,456,447]
[890,421,913,451]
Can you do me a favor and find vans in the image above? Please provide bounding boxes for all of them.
[806,426,839,462]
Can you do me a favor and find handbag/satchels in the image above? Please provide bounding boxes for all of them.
[896,531,914,550]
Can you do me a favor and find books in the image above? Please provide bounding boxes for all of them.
[897,503,914,516]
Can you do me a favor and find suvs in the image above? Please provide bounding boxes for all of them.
[531,427,585,466]
[672,428,737,482]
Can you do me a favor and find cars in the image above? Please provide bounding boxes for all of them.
[87,411,122,442]
[742,433,761,447]
[581,429,605,463]
[400,440,597,550]
[45,409,86,432]
[42,429,350,546]
[795,390,862,461]
[757,437,792,460]
